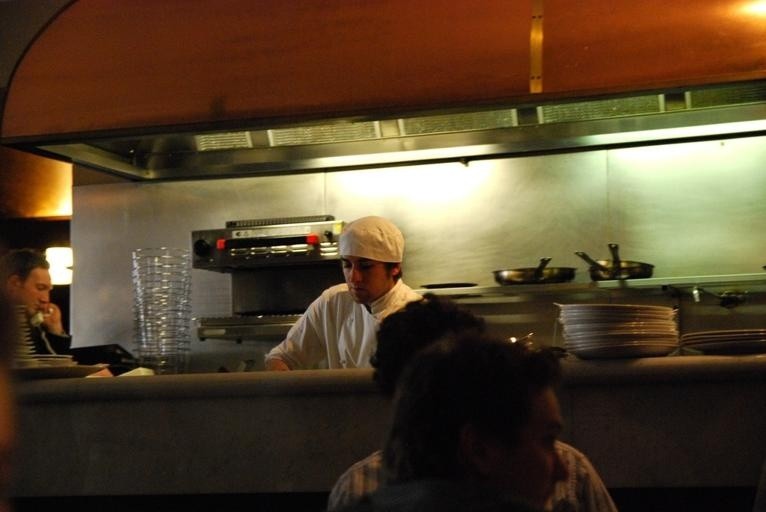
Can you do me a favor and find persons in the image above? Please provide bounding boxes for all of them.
[0,246,114,378]
[339,331,572,511]
[261,214,425,372]
[325,291,618,512]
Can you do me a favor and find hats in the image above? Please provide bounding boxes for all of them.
[340,216,404,263]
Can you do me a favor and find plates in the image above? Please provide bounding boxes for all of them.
[5,365,110,380]
[552,300,766,361]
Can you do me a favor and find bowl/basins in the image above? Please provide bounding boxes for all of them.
[127,245,197,373]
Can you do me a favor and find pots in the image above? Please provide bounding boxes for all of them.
[490,258,576,287]
[573,244,654,280]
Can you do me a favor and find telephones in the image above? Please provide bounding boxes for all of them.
[30,308,53,326]
